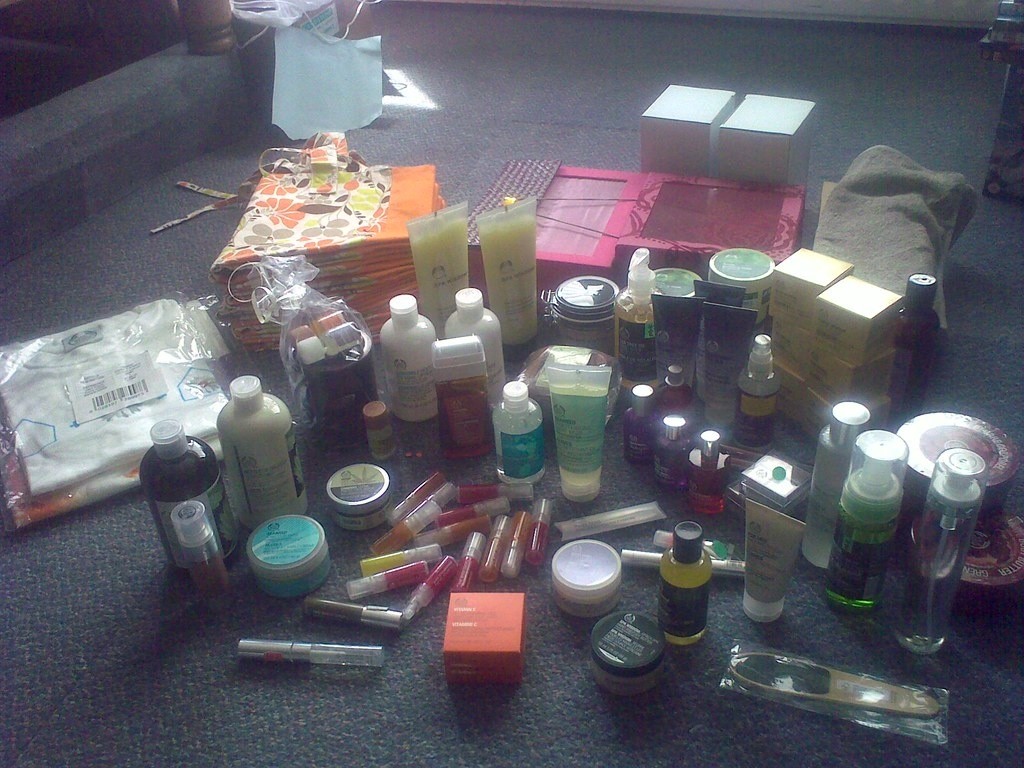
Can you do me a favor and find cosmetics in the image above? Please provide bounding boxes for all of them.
[138,81,1024,697]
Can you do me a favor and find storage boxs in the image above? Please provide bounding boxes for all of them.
[442,593,530,687]
[465,85,903,442]
[725,450,811,523]
[240,0,374,67]
